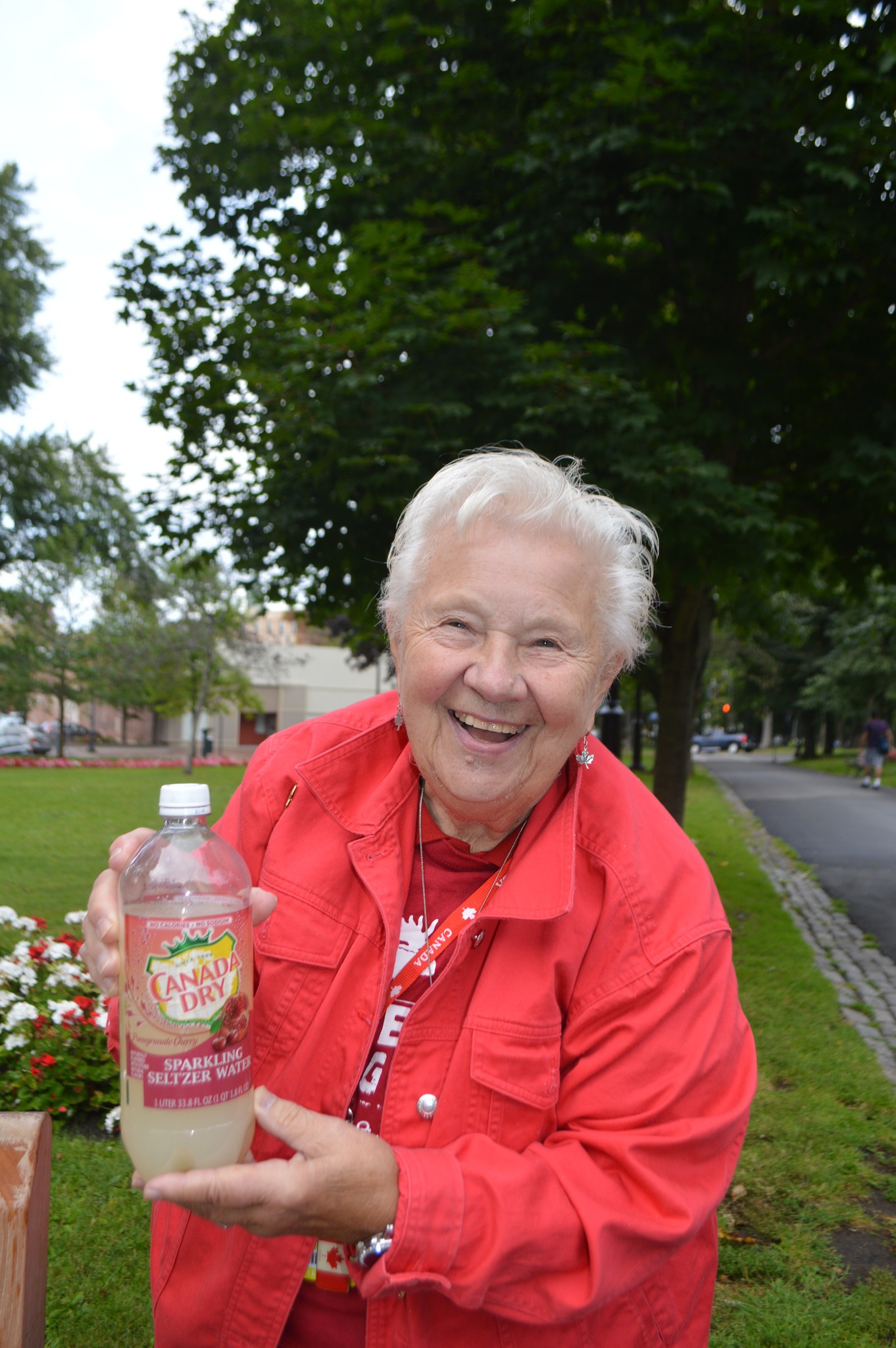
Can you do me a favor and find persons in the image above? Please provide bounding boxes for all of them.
[203,730,212,757]
[855,712,893,790]
[81,444,756,1348]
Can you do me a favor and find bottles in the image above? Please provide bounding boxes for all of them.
[118,783,256,1184]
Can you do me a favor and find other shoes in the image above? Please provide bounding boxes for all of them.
[862,777,871,787]
[873,779,880,789]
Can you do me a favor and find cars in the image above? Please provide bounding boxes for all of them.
[41,721,102,746]
[690,725,755,756]
[0,716,51,756]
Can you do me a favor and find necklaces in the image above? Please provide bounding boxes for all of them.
[419,784,529,987]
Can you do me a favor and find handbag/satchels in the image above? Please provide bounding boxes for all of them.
[879,743,889,754]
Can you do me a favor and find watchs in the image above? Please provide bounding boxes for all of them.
[356,1223,394,1267]
